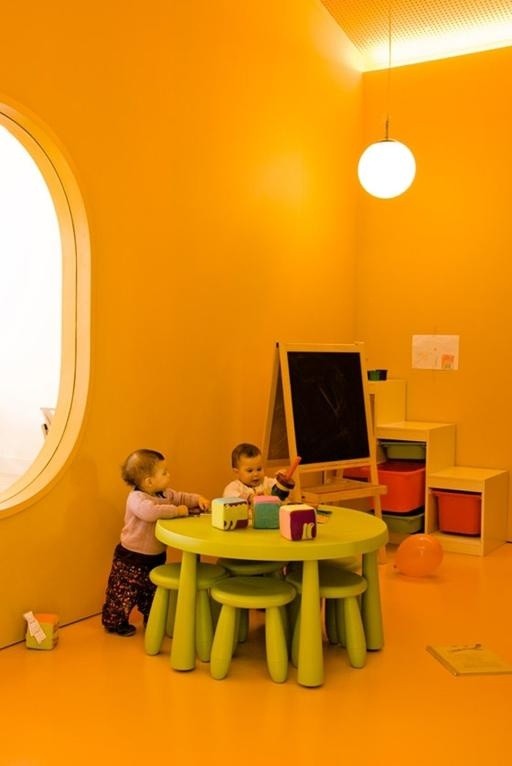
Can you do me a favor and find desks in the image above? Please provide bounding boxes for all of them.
[155,500,390,688]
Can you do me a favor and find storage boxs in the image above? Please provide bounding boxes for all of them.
[432,490,481,535]
[362,460,425,513]
[332,465,372,482]
[378,441,426,459]
[372,505,425,535]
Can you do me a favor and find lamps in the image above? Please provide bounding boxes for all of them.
[358,0,417,199]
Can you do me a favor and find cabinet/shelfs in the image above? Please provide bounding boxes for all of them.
[366,378,507,557]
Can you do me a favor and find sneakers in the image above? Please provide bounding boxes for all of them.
[105,622,137,637]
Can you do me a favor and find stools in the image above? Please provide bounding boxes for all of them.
[209,575,297,682]
[285,566,369,668]
[286,555,361,648]
[144,561,230,662]
[215,558,289,643]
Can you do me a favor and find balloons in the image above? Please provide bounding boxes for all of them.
[392,533,444,575]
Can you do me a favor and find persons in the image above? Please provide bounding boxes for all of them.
[100,449,211,636]
[221,442,279,506]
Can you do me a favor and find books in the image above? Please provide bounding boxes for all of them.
[425,640,509,678]
